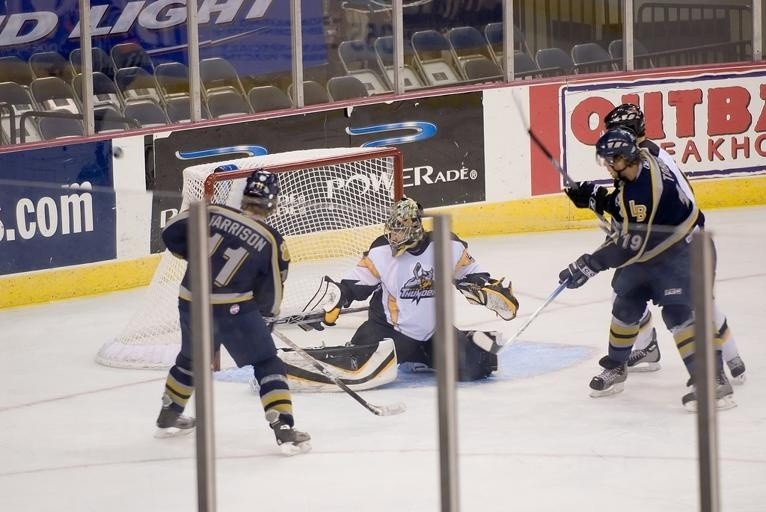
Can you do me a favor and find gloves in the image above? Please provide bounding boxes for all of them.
[563,179,606,217]
[298,277,352,336]
[557,253,603,288]
[458,273,523,325]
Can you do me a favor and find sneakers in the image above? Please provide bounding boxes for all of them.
[157,407,196,429]
[589,362,626,390]
[687,376,694,388]
[598,328,661,364]
[682,367,734,406]
[271,420,311,446]
[725,357,745,377]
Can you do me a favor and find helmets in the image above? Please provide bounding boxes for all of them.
[603,104,646,138]
[385,198,424,259]
[243,170,279,212]
[595,129,641,162]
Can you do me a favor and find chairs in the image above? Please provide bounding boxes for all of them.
[374,36,429,91]
[29,76,82,116]
[410,29,468,86]
[164,96,211,124]
[38,109,86,143]
[206,90,254,121]
[0,82,38,116]
[571,42,617,73]
[501,52,542,78]
[609,37,652,71]
[338,38,393,95]
[120,102,171,130]
[70,47,116,75]
[448,25,501,81]
[287,79,331,107]
[326,76,369,102]
[28,51,74,82]
[110,42,155,72]
[535,46,577,78]
[198,57,247,100]
[154,62,206,109]
[113,66,165,107]
[72,71,123,111]
[92,106,130,133]
[249,86,293,114]
[0,54,34,88]
[484,21,541,78]
[464,59,504,82]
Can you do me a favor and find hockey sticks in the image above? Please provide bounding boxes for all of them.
[467,232,619,355]
[272,328,407,417]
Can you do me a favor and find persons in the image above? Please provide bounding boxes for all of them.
[559,125,738,412]
[565,104,745,384]
[252,197,519,394]
[154,170,312,456]
